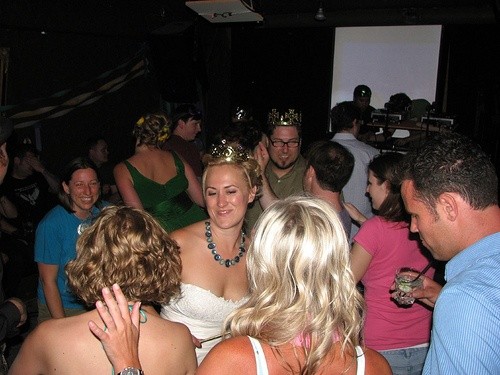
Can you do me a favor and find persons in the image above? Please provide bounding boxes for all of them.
[2,139,62,265]
[81,136,121,206]
[334,84,378,142]
[33,159,113,323]
[7,205,198,375]
[165,105,268,183]
[344,152,436,375]
[87,196,394,375]
[322,102,382,244]
[387,92,442,152]
[0,113,37,375]
[243,109,315,238]
[389,130,500,375]
[113,115,209,231]
[252,142,351,241]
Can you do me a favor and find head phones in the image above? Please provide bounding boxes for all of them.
[405,105,412,112]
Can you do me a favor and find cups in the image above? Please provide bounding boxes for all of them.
[393,266,424,305]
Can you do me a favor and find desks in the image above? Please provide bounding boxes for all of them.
[364,119,447,156]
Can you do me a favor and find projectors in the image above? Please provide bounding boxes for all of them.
[185,0,263,23]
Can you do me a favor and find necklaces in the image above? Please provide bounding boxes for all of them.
[205,218,247,267]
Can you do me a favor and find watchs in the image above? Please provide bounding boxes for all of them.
[118,367,145,375]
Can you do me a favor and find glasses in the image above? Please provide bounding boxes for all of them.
[270,138,300,148]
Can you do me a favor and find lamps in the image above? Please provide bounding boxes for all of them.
[316,7,325,19]
